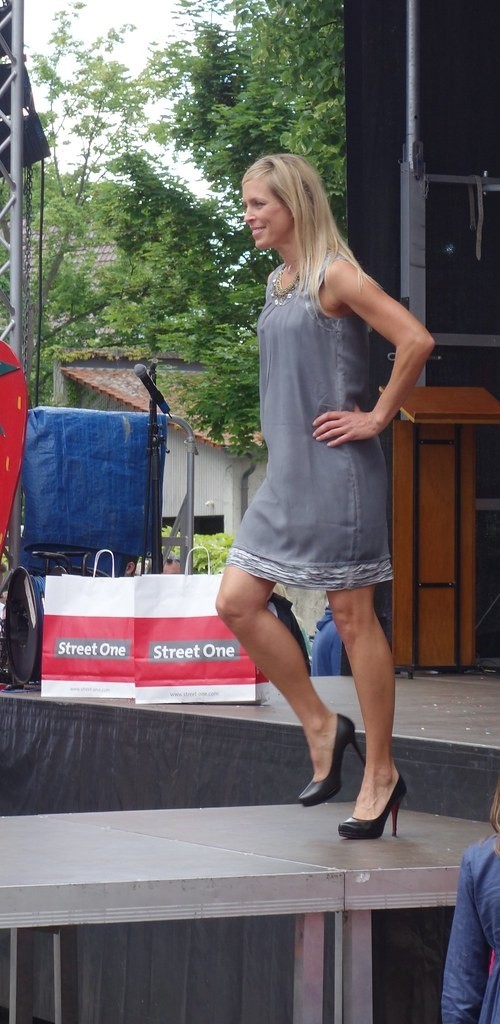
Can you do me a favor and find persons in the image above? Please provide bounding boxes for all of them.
[123,555,138,577]
[163,557,181,574]
[441,778,500,1024]
[265,583,343,676]
[215,152,434,840]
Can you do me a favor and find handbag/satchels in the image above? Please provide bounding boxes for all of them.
[134,546,270,705]
[40,547,134,698]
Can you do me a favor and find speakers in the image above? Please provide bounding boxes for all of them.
[0,5,52,176]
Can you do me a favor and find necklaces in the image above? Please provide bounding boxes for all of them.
[271,266,300,305]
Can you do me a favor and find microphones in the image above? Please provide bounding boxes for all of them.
[134,364,173,420]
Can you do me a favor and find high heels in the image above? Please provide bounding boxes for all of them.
[338,773,406,839]
[299,714,365,807]
